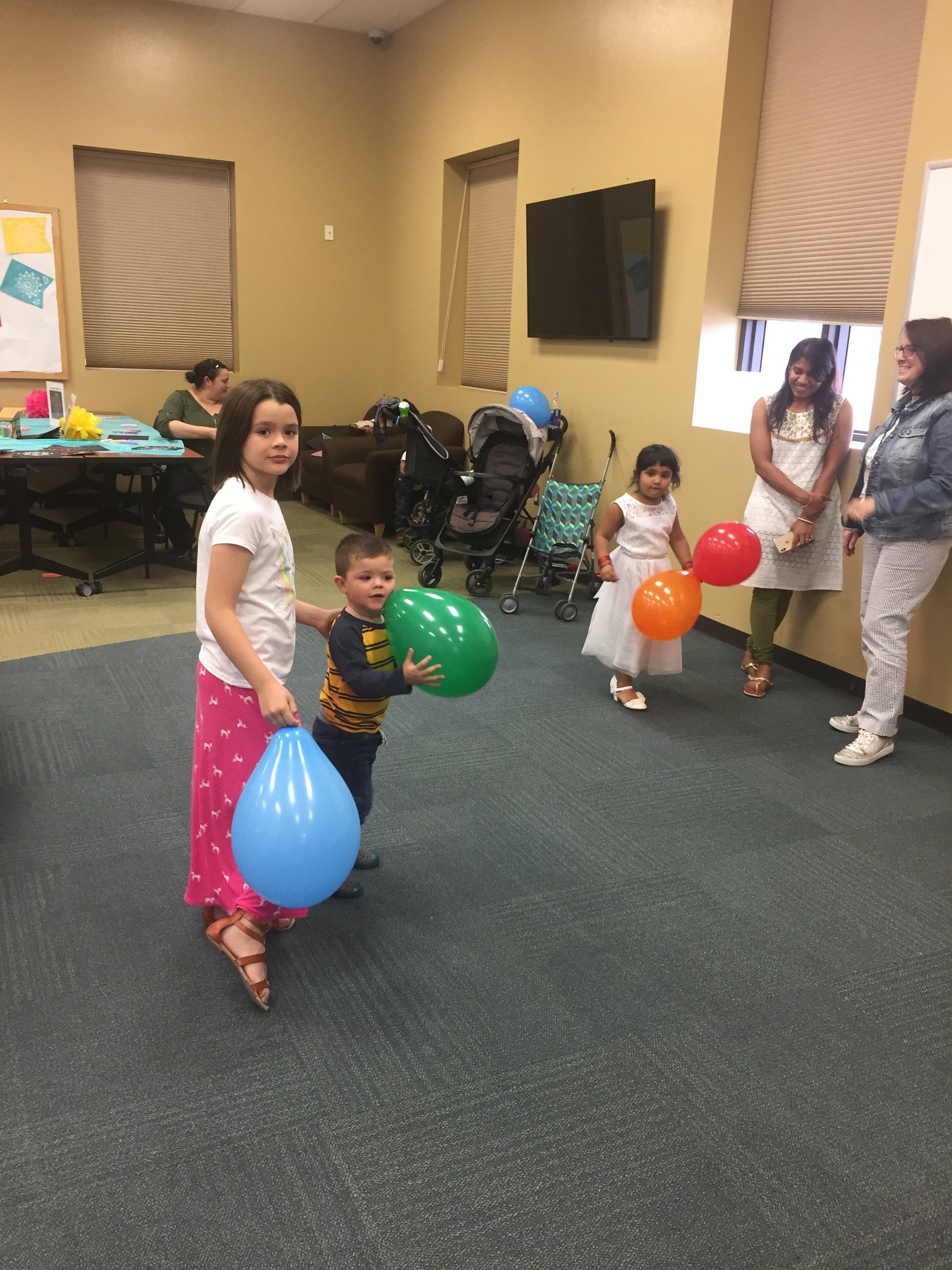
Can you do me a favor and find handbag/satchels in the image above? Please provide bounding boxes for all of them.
[363,396,421,435]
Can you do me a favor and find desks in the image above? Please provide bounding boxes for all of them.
[0,411,204,596]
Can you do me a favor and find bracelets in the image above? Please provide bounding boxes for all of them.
[797,517,814,524]
[684,565,692,571]
[600,561,612,568]
[682,561,692,568]
[801,492,812,507]
[598,556,611,564]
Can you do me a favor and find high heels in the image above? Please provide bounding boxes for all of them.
[610,674,647,711]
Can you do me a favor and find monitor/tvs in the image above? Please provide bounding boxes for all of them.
[526,179,655,340]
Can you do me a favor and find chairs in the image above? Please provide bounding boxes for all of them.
[151,488,211,571]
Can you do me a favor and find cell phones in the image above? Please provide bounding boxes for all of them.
[773,531,813,554]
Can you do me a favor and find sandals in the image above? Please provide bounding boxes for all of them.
[206,905,272,1012]
[202,898,294,931]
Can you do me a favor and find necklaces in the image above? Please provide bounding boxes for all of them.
[869,411,897,464]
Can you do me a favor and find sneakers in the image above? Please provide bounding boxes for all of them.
[829,714,864,733]
[171,534,198,557]
[834,730,895,765]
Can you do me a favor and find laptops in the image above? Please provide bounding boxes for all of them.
[46,381,65,435]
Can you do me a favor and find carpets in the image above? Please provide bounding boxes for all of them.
[0,588,952,1270]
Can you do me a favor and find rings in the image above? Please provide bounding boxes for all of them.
[805,539,808,540]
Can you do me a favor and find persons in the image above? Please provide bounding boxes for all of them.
[153,358,231,557]
[184,380,344,1012]
[829,316,952,766]
[393,425,432,547]
[311,533,444,899]
[741,337,854,692]
[581,445,703,709]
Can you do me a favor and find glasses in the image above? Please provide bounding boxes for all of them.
[894,342,917,359]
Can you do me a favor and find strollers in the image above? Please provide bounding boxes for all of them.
[403,403,569,597]
[500,428,615,621]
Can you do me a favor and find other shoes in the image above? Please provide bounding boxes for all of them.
[353,850,381,869]
[397,531,407,547]
[336,878,362,897]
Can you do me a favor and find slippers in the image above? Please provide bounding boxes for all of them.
[744,676,770,698]
[740,662,759,675]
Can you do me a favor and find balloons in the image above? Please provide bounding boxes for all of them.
[631,570,702,640]
[509,386,552,428]
[384,587,498,698]
[692,522,762,586]
[231,730,361,909]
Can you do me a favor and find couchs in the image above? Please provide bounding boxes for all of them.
[266,393,416,516]
[321,410,467,542]
